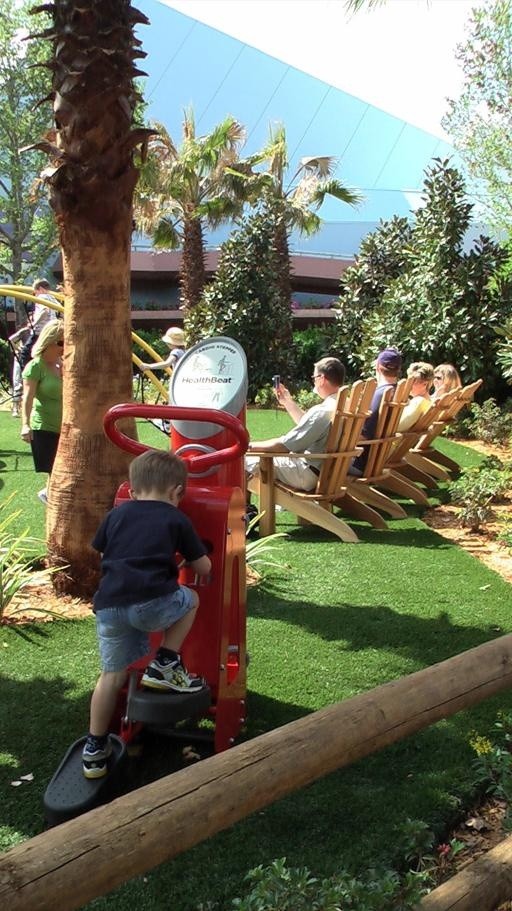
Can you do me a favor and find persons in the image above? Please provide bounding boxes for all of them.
[18,278,64,371]
[429,364,462,403]
[385,360,437,464]
[20,317,68,508]
[81,452,211,785]
[140,326,189,372]
[8,311,37,417]
[240,356,352,537]
[345,349,404,477]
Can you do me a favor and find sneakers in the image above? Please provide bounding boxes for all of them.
[140,655,206,693]
[153,419,171,434]
[13,407,19,418]
[82,735,112,780]
[36,488,47,504]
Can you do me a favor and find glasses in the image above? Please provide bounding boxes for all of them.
[434,375,442,380]
[54,340,63,347]
[311,373,327,382]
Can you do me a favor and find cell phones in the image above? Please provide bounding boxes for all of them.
[276,376,280,391]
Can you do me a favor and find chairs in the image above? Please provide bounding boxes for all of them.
[350,374,482,544]
[237,373,387,544]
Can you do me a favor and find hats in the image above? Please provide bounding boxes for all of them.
[375,350,401,370]
[162,327,186,349]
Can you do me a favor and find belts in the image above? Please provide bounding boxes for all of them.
[305,462,320,476]
